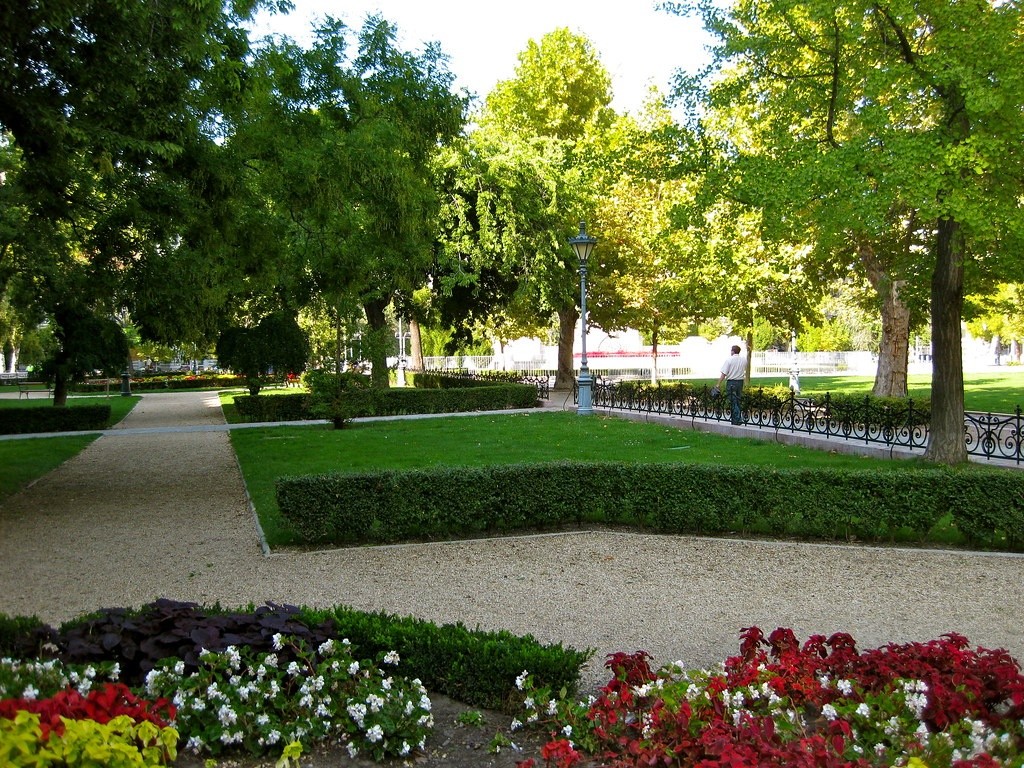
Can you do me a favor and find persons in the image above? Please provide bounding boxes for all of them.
[715,345,746,426]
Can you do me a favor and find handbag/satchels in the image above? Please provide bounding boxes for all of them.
[710,386,720,400]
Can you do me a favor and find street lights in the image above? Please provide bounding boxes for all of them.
[569,221,597,417]
